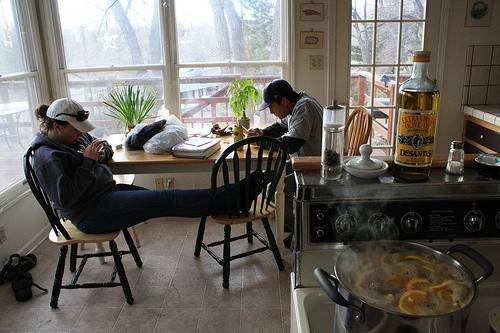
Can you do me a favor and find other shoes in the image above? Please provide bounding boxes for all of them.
[283,232,294,249]
[4,253,37,283]
[11,265,47,302]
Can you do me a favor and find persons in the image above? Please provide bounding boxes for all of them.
[248,79,324,157]
[34,98,272,234]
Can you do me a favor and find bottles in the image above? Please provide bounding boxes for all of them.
[445,141,464,174]
[321,99,346,182]
[392,51,441,182]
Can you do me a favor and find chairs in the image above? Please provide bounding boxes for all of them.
[341,103,372,158]
[194,135,288,290]
[22,146,143,309]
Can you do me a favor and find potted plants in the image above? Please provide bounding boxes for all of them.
[99,80,162,131]
[227,77,260,131]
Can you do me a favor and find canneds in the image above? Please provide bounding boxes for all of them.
[233,125,243,149]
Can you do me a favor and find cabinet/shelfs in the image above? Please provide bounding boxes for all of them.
[461,116,500,157]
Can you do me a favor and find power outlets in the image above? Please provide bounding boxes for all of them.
[165,177,174,189]
[154,178,163,191]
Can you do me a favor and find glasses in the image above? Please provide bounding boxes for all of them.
[56,111,89,122]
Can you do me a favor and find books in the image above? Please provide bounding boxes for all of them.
[171,137,222,160]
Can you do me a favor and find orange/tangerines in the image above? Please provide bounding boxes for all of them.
[398,255,459,316]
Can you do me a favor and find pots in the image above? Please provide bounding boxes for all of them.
[314,240,494,333]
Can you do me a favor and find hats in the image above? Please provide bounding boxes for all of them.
[260,80,293,111]
[46,98,96,133]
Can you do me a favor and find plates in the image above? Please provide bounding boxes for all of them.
[474,156,500,166]
[343,157,388,179]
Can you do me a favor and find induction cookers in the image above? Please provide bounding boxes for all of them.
[290,154,500,333]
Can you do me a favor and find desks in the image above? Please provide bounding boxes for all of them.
[90,130,289,265]
